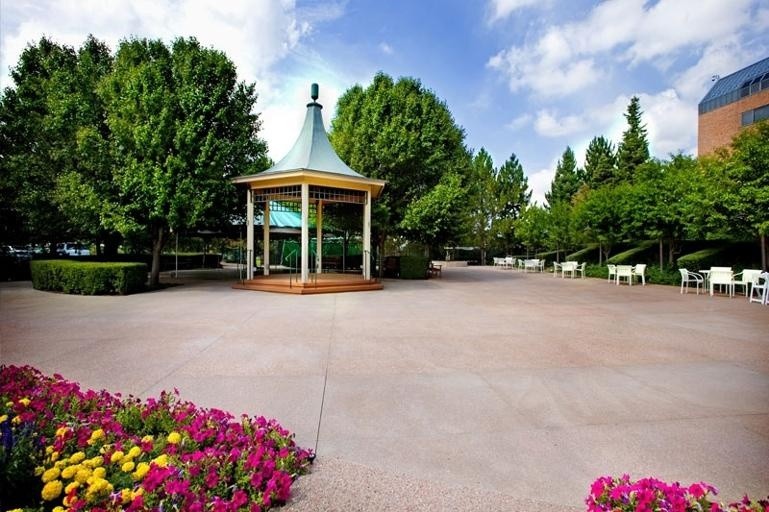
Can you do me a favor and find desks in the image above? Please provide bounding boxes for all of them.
[698,270,734,293]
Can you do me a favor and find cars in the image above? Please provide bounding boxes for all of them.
[3,242,91,256]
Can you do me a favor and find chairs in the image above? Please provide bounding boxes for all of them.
[492,255,587,280]
[678,268,705,296]
[427,262,442,278]
[607,264,616,285]
[616,265,632,286]
[733,269,763,298]
[707,267,735,298]
[632,263,647,286]
